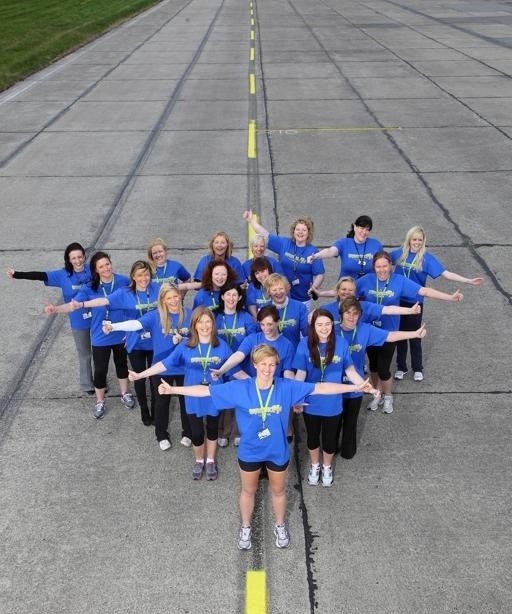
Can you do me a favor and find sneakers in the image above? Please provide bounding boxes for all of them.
[307,463,321,485]
[93,400,105,419]
[414,372,423,381]
[394,371,407,380]
[121,392,135,409]
[237,526,252,550]
[322,464,333,486]
[274,524,290,548]
[382,396,393,413]
[142,413,240,481]
[367,398,384,410]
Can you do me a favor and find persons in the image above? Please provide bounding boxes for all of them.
[158,343,371,549]
[7,209,483,487]
